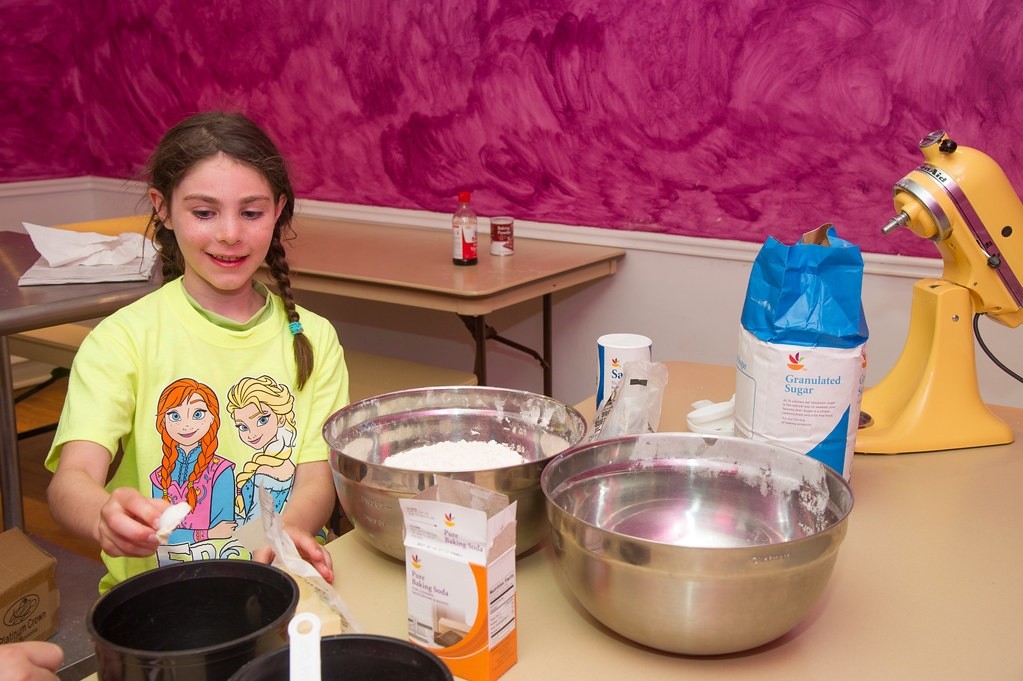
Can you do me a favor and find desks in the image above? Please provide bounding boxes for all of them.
[257,214,628,399]
[4,212,172,377]
[297,361,1020,681]
[0,230,171,536]
[342,347,480,401]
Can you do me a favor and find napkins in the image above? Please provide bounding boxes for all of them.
[17,220,163,268]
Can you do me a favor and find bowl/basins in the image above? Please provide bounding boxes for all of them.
[225,630,457,681]
[319,385,586,568]
[85,557,302,681]
[538,433,857,655]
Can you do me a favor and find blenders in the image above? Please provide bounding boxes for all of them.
[850,128,1022,455]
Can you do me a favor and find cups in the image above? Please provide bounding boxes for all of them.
[595,333,653,417]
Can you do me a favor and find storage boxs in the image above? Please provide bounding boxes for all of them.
[0,527,61,645]
[398,472,515,681]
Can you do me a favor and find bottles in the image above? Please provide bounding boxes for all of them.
[452,192,478,265]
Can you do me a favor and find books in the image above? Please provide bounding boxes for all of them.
[17,221,163,287]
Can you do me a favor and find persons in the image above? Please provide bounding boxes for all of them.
[0,640,63,681]
[43,111,350,597]
